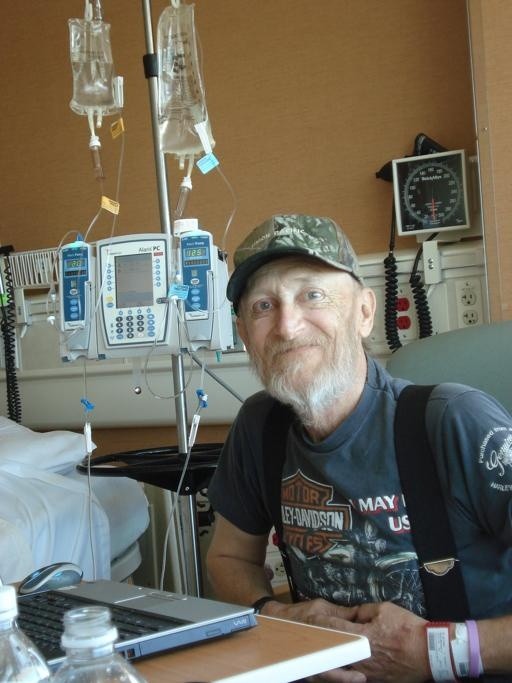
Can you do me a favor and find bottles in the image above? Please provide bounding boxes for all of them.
[0,583,148,682]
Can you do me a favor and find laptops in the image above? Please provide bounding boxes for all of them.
[15,579,257,674]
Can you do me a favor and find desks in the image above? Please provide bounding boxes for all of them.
[8,580,371,682]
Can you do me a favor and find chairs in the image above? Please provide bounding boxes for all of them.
[386,320,512,416]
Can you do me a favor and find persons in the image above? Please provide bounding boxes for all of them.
[204,213,510,682]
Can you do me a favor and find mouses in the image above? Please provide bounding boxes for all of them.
[18,562,83,594]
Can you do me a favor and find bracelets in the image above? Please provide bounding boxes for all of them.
[423,621,458,683]
[246,591,276,615]
[464,618,480,679]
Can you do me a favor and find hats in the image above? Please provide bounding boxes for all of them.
[226,213,364,315]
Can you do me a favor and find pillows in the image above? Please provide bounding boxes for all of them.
[0,415,98,476]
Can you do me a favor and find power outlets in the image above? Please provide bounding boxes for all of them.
[456,279,483,329]
[395,285,417,342]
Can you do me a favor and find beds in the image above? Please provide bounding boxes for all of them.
[0,463,151,584]
